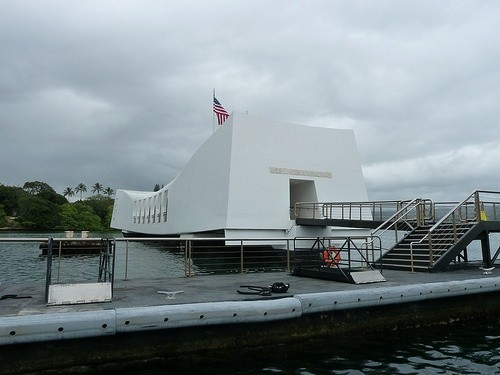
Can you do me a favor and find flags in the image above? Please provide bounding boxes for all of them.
[213,97,230,125]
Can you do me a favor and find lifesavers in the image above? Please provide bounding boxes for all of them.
[323,247,341,265]
